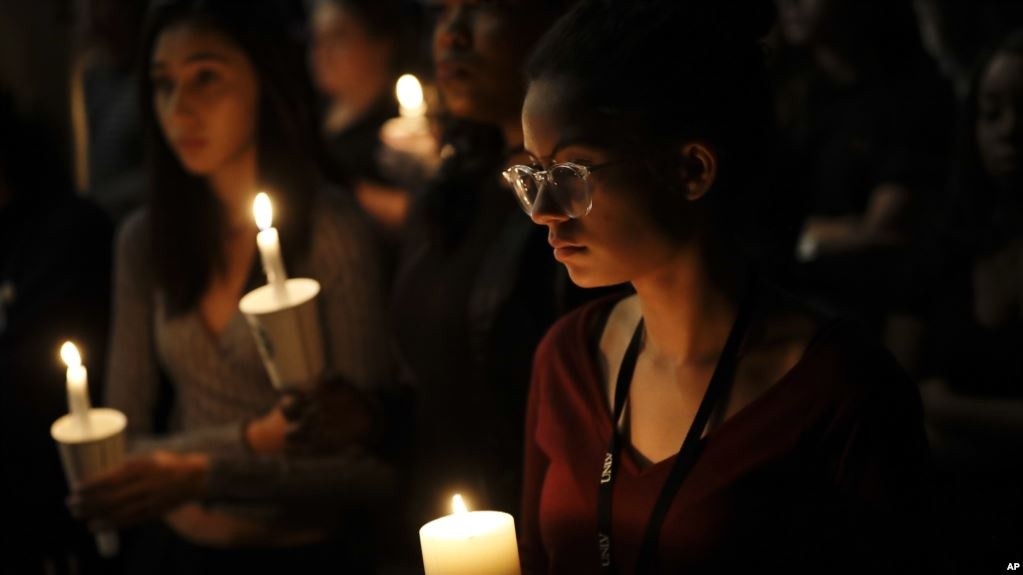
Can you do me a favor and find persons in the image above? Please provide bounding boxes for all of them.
[0,0,1023,575]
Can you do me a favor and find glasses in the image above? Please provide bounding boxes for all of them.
[501,149,678,219]
